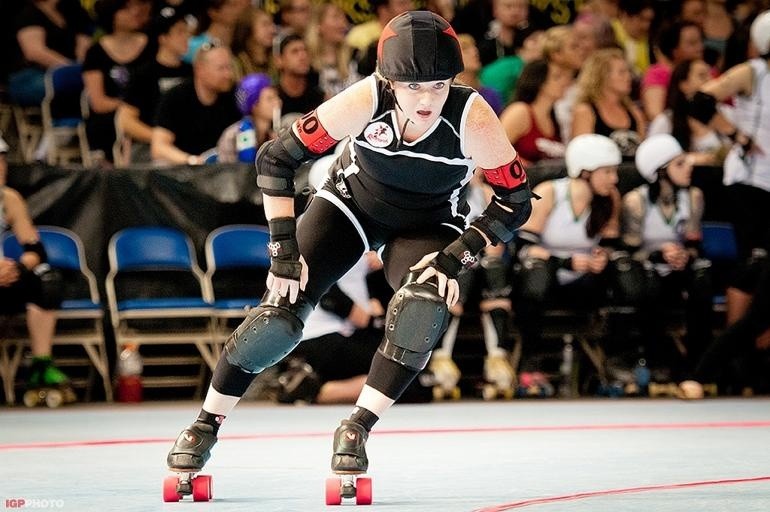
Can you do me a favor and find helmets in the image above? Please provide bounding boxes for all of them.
[234,74,276,115]
[377,10,464,82]
[565,134,684,183]
[749,8,770,55]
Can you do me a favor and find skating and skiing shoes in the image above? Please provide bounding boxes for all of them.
[164,421,217,502]
[428,348,516,401]
[325,418,372,505]
[23,355,76,409]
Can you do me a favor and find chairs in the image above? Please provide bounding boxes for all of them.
[460,215,740,399]
[1,62,116,169]
[203,225,271,360]
[106,226,219,399]
[1,227,112,404]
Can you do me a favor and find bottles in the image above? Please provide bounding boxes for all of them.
[117,342,142,403]
[237,118,257,162]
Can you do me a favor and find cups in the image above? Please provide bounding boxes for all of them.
[557,351,580,398]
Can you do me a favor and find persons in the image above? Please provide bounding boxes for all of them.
[165,9,532,476]
